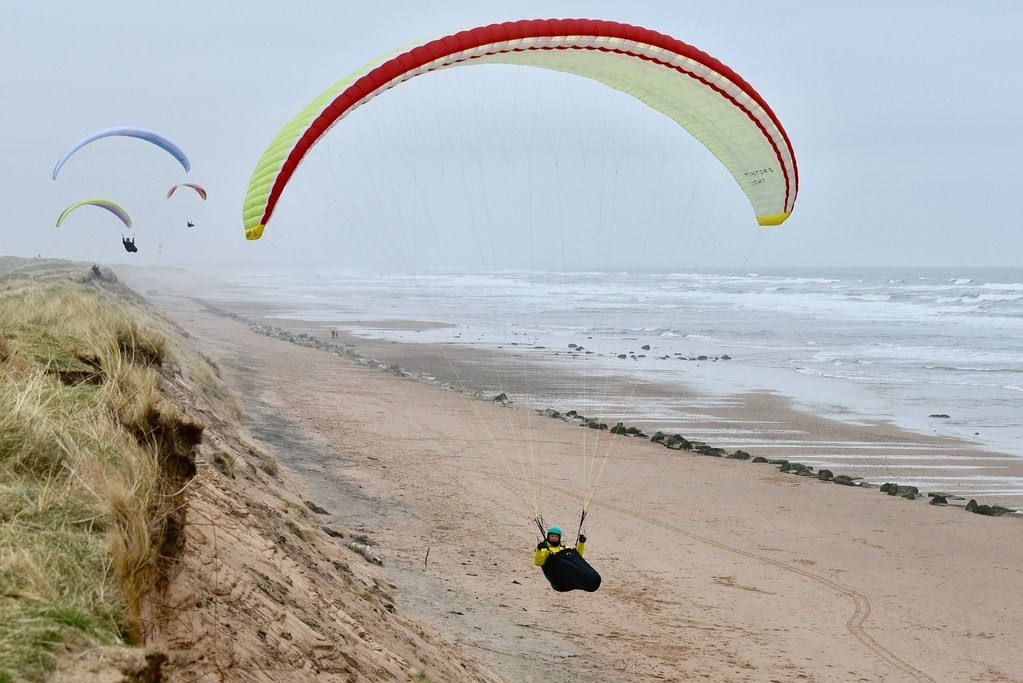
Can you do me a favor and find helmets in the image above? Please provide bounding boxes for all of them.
[547,526,562,536]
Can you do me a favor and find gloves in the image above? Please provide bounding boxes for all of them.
[579,534,586,543]
[538,542,546,550]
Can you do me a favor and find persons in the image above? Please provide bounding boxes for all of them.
[121,236,138,252]
[533,526,601,592]
[188,222,195,227]
